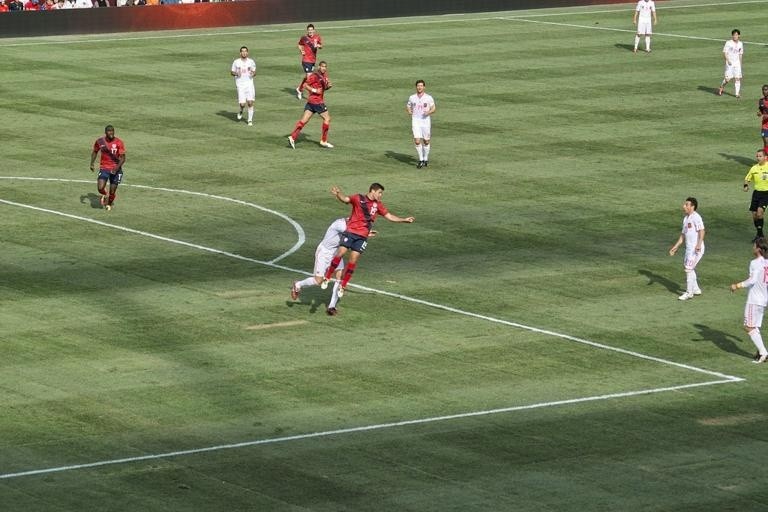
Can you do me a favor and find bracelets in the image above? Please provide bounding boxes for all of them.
[743,184,747,188]
[311,88,316,93]
[735,281,742,288]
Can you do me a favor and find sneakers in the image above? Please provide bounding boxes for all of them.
[320,140,333,147]
[751,350,768,364]
[105,204,114,211]
[296,87,302,100]
[287,136,295,149]
[751,236,764,243]
[679,291,693,300]
[291,278,345,316]
[736,95,740,99]
[417,160,424,169]
[101,195,107,206]
[237,110,243,120]
[633,48,638,53]
[693,289,701,295]
[719,87,724,95]
[248,121,252,126]
[645,48,652,53]
[423,161,429,167]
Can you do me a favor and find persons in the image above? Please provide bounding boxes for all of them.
[288,60,333,149]
[320,183,415,297]
[405,79,435,169]
[729,237,767,362]
[669,196,705,300]
[291,217,379,315]
[718,29,743,97]
[295,23,323,100]
[0,0,234,11]
[756,85,767,160]
[742,149,767,243]
[231,46,256,125]
[90,125,127,211]
[632,0,656,52]
